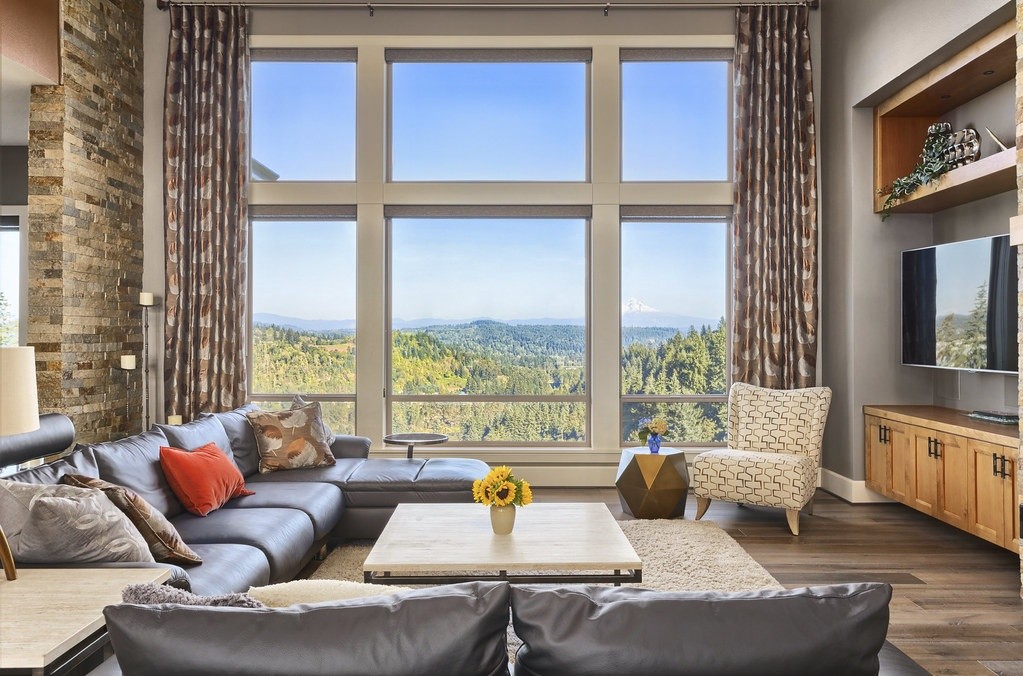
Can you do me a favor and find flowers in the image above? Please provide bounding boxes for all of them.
[638,417,667,447]
[472,465,533,507]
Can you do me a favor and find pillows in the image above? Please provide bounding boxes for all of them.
[246,401,336,474]
[102,582,510,676]
[160,442,255,516]
[511,579,893,676]
[64,472,203,565]
[0,478,156,564]
[290,394,336,447]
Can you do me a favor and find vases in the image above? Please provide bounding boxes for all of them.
[648,433,661,453]
[490,502,516,535]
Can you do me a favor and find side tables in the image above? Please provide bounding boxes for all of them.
[0,568,171,676]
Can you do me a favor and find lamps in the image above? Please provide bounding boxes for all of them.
[0,345,40,581]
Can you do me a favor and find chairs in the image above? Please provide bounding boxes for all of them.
[693,381,832,536]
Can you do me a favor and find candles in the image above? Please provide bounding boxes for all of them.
[140,292,153,305]
[121,354,136,369]
[168,408,182,425]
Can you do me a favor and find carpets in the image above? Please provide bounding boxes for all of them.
[307,519,786,664]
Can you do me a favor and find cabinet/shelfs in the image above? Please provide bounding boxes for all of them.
[865,413,1019,554]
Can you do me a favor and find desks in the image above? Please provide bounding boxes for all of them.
[615,446,691,520]
[362,503,643,590]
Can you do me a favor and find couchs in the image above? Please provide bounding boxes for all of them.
[0,408,496,596]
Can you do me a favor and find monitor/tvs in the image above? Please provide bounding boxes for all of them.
[900,233,1018,374]
